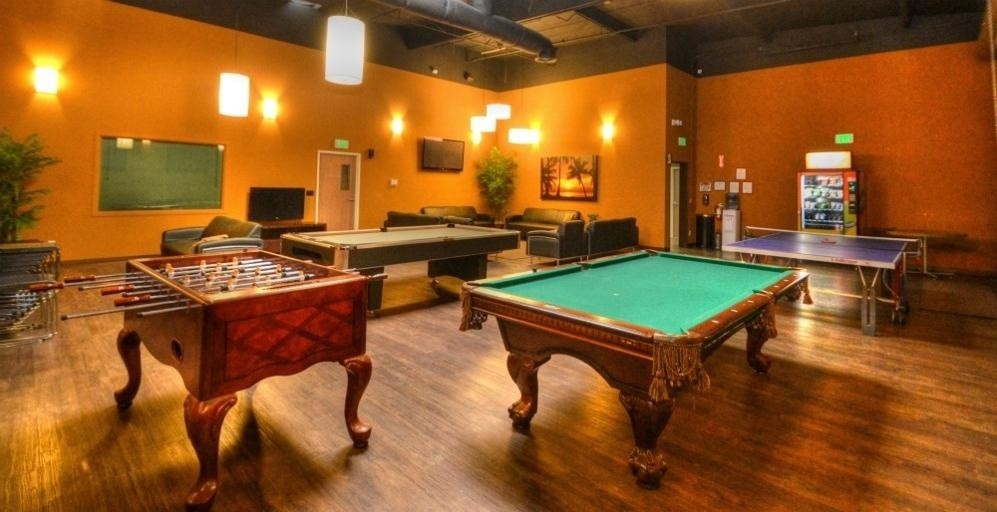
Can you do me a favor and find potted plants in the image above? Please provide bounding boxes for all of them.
[475,148,518,229]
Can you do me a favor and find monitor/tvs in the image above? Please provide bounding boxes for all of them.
[422,137,465,170]
[247,187,305,222]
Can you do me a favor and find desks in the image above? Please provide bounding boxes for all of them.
[278,223,521,311]
[883,224,969,282]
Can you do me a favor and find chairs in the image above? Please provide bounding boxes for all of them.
[158,215,263,255]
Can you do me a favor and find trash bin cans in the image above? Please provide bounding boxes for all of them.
[696,214,715,249]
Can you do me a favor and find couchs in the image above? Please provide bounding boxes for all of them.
[382,206,638,266]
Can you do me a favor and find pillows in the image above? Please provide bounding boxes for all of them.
[191,233,229,249]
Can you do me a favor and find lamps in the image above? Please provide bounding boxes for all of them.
[217,0,250,118]
[468,42,543,145]
[322,1,365,86]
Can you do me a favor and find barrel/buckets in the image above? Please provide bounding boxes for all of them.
[726,192,738,210]
